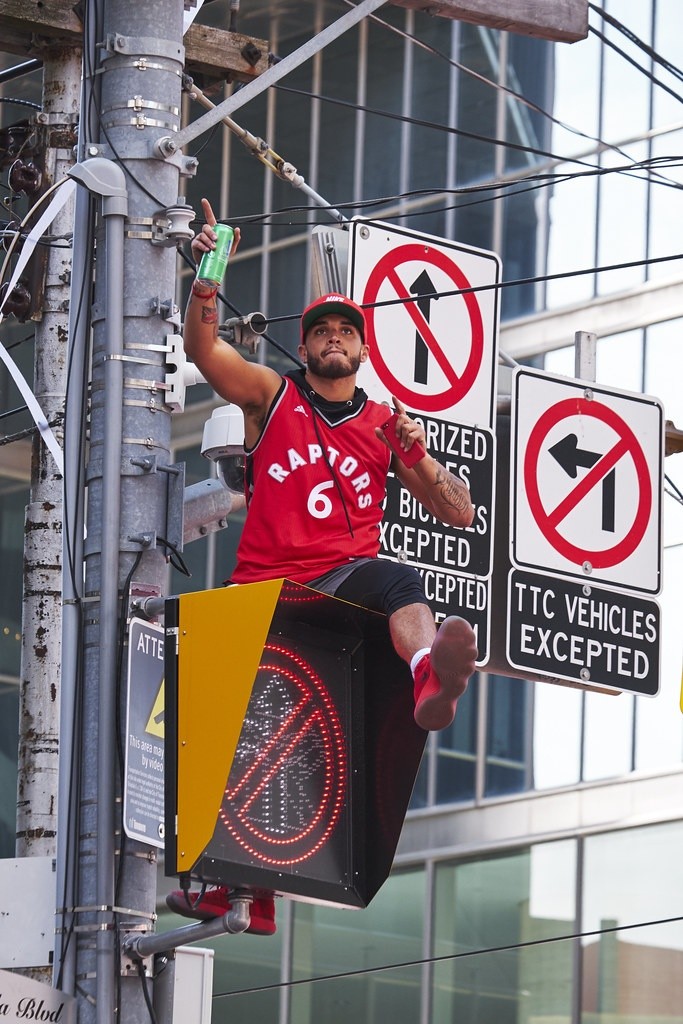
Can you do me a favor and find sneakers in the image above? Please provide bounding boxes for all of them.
[166,886,280,935]
[413,612,479,731]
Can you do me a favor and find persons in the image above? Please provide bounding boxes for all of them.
[165,198,477,934]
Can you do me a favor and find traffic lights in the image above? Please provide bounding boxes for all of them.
[163,579,434,911]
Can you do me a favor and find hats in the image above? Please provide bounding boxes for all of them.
[300,292,367,346]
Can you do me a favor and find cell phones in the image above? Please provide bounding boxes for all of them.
[381,413,426,469]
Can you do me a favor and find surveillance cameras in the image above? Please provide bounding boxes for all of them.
[200,400,247,495]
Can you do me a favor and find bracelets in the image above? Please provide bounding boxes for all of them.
[192,282,217,301]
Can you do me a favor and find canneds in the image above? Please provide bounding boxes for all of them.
[196,224,235,287]
